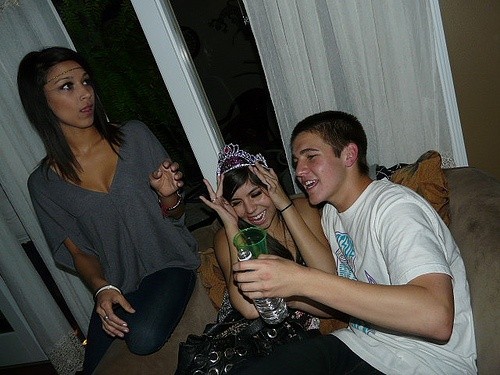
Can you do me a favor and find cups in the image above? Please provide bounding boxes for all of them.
[233,228,269,261]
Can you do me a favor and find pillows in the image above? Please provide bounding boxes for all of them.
[374,149,450,227]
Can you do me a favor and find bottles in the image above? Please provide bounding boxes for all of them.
[237,248,288,326]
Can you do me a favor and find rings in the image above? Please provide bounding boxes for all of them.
[103,316,109,321]
[211,197,217,202]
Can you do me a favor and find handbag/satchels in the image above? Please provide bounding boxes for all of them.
[176,308,309,375]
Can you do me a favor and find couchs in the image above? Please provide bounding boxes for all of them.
[91,165,500,375]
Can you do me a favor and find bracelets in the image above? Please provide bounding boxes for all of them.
[280,202,293,212]
[158,196,181,211]
[94,285,123,300]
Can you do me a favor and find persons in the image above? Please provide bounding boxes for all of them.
[232,110,478,375]
[174,143,338,375]
[16,47,201,375]
